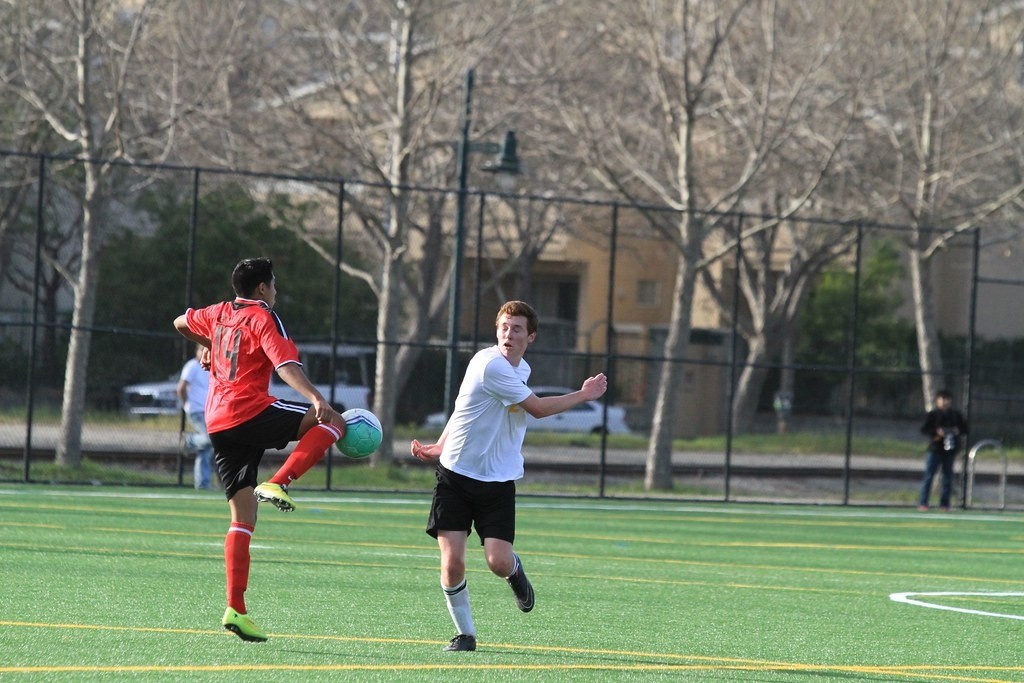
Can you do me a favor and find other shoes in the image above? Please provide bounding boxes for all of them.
[919,505,928,512]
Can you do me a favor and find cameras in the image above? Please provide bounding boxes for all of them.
[942,428,955,450]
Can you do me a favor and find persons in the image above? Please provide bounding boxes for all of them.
[410,300,607,651]
[916,390,968,511]
[174,342,219,491]
[173,257,345,643]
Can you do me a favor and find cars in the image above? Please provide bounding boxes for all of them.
[422,385,633,436]
[118,369,374,419]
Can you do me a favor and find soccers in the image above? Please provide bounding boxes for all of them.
[334,408,383,460]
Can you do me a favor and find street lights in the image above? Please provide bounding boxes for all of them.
[441,64,525,430]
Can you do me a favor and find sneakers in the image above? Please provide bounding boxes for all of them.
[443,634,476,652]
[221,606,268,642]
[253,482,296,512]
[506,552,535,612]
